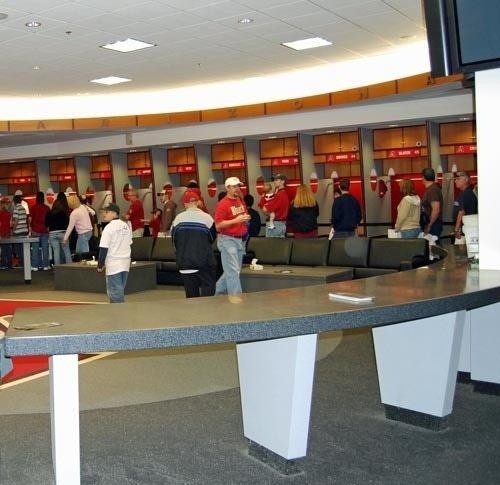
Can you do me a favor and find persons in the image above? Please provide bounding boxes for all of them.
[157,189,177,232]
[454,171,478,251]
[30,192,51,271]
[264,174,290,238]
[394,178,422,239]
[63,195,96,255]
[121,183,145,238]
[170,187,217,298]
[328,178,362,239]
[418,168,444,260]
[258,183,276,229]
[214,176,251,295]
[244,194,261,237]
[0,201,12,269]
[12,196,29,268]
[15,190,29,214]
[334,188,341,197]
[289,184,320,239]
[46,198,73,264]
[97,203,133,304]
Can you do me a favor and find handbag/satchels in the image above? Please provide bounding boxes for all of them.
[84,204,99,238]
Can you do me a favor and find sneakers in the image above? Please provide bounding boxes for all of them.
[30,265,55,271]
[0,265,24,270]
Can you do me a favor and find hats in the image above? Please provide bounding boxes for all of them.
[157,190,167,196]
[225,176,243,189]
[182,191,199,203]
[101,203,120,213]
[272,174,287,181]
[451,170,469,178]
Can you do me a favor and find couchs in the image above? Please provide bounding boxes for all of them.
[244,233,430,280]
[130,237,176,284]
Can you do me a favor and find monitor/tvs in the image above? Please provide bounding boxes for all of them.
[423,0,499,77]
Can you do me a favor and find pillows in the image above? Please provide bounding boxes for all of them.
[328,236,370,268]
[131,235,154,261]
[290,237,330,267]
[370,238,425,267]
[154,237,177,262]
[246,236,293,266]
